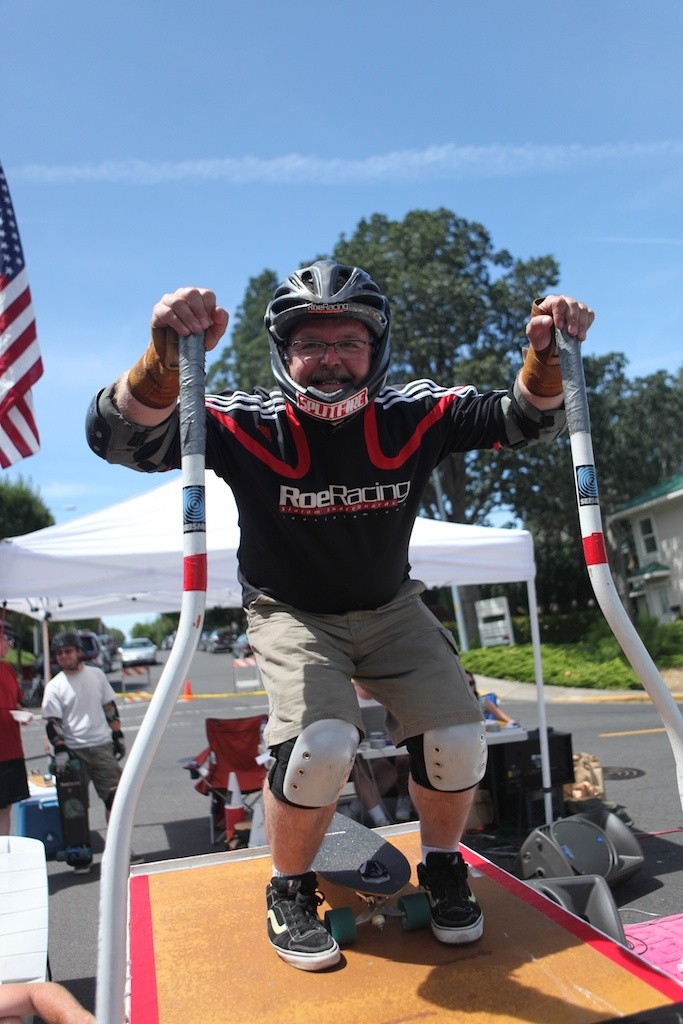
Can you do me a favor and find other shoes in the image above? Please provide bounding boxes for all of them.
[75,858,92,874]
[129,854,142,864]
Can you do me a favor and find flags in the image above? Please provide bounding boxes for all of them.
[0,162,44,471]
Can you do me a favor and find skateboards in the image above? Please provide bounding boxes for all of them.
[49,759,93,873]
[309,810,431,947]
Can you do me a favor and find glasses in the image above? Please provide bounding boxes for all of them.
[286,339,374,360]
[55,649,73,656]
[3,634,15,643]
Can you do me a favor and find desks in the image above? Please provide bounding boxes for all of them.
[357,728,529,830]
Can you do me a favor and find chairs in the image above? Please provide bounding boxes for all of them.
[0,835,51,1024]
[184,714,270,844]
[361,706,388,735]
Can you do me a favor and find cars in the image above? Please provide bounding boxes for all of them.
[161,626,253,659]
[72,632,117,673]
[118,637,159,666]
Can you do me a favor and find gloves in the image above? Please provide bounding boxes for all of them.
[54,744,71,773]
[112,730,126,760]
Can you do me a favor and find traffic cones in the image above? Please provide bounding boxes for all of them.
[182,678,198,702]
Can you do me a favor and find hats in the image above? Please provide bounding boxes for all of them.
[0,621,20,638]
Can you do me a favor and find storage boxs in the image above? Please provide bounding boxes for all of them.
[14,775,65,860]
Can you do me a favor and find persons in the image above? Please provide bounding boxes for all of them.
[40,631,144,874]
[86,262,595,971]
[466,669,516,722]
[0,636,34,836]
[0,981,96,1024]
[354,682,411,826]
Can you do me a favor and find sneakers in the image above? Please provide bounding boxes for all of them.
[416,851,484,945]
[265,871,341,971]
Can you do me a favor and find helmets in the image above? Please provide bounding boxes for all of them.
[51,631,83,652]
[264,260,391,427]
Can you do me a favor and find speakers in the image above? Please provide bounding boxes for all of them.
[519,808,645,888]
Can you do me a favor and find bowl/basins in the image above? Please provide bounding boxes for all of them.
[10,710,33,723]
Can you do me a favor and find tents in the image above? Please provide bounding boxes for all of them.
[1,470,553,825]
